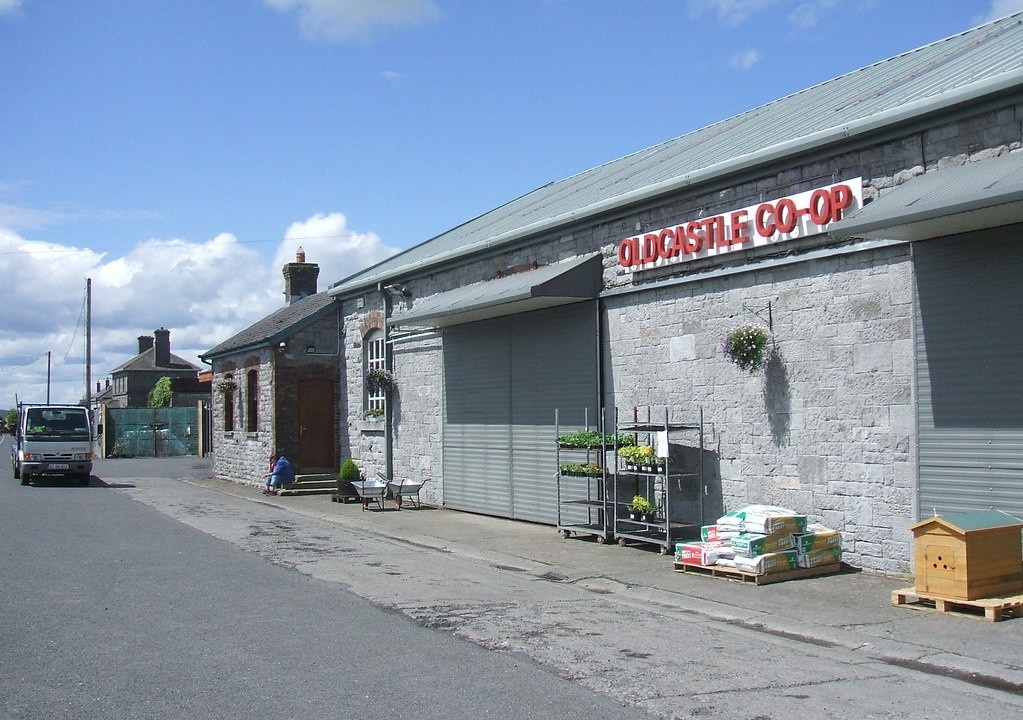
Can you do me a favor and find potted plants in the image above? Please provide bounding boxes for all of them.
[364,408,384,423]
[337,459,366,494]
[556,430,634,450]
[560,462,610,478]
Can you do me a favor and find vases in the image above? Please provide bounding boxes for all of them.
[626,461,666,474]
[629,512,654,523]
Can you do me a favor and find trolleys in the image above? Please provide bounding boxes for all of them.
[350,476,392,512]
[388,478,431,511]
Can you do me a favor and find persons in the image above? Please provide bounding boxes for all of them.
[262,453,295,496]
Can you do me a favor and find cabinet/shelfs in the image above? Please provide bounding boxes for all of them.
[555,440,639,544]
[890,586,1022,623]
[614,422,703,555]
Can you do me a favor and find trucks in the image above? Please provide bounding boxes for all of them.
[10,401,93,486]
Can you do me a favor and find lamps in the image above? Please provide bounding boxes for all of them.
[384,284,412,299]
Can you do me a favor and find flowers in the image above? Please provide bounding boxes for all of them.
[720,321,773,373]
[364,367,397,393]
[627,495,657,513]
[615,445,664,464]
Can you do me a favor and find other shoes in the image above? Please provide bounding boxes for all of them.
[262,489,270,494]
[267,492,275,496]
